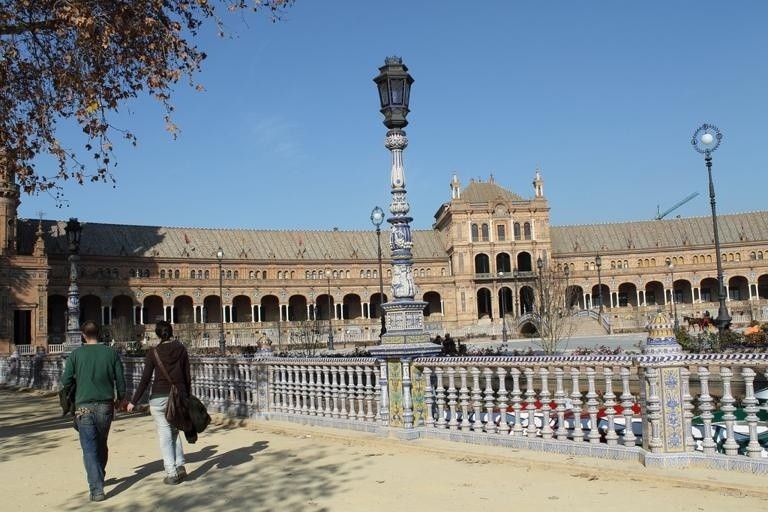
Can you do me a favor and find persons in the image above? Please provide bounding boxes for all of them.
[703,318,708,332]
[432,333,469,357]
[125,319,191,484]
[62,319,127,503]
[706,310,710,318]
[744,320,760,335]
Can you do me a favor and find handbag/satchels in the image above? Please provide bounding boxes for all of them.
[166,386,192,431]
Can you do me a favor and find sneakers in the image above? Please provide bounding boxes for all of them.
[90,494,104,501]
[164,471,186,484]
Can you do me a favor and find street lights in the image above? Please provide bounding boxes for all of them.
[61,217,84,352]
[370,205,387,340]
[323,267,335,351]
[690,121,734,340]
[216,245,227,358]
[563,264,571,315]
[595,251,605,316]
[498,271,508,342]
[668,263,680,332]
[366,53,443,359]
[512,267,519,320]
[536,254,545,319]
